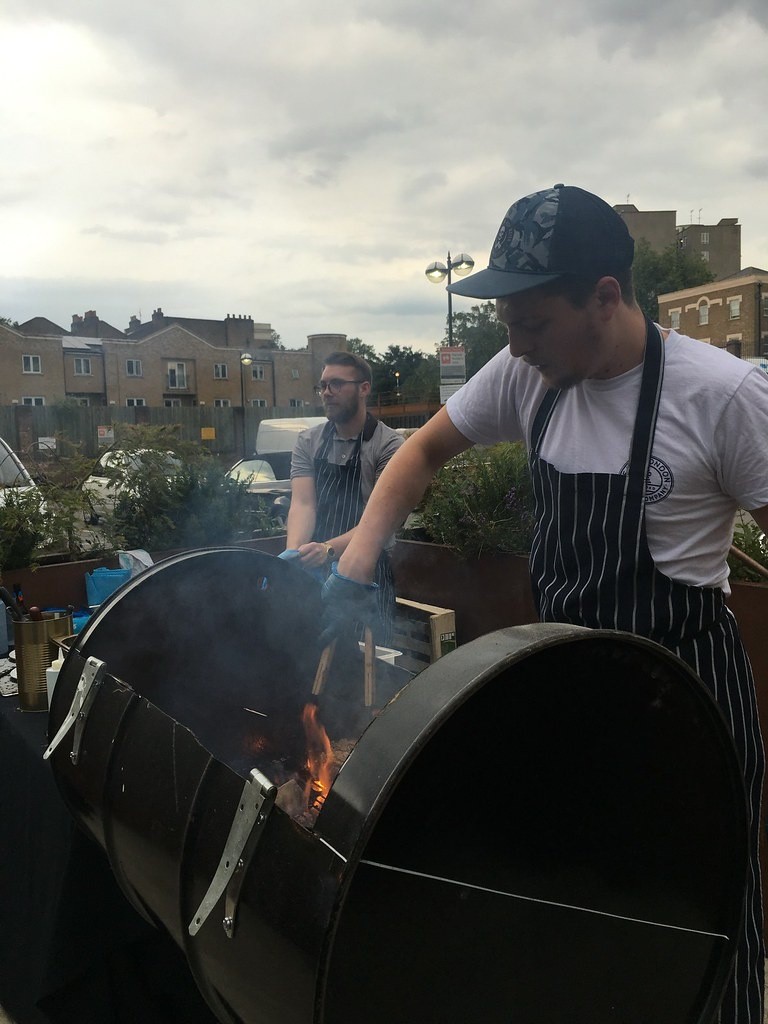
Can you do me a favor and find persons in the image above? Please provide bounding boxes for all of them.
[318,182,768,1024]
[277,351,406,649]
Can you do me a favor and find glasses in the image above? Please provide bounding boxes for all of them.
[313,380,367,398]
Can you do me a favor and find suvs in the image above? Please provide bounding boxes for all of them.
[80,449,207,526]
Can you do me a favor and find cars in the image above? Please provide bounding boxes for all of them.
[224,452,292,524]
[0,437,69,553]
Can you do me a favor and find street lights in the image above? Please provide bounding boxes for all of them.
[425,251,474,346]
[239,352,252,407]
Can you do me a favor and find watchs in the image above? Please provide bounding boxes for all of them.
[321,543,335,561]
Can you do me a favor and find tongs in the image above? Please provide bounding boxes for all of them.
[300,624,377,732]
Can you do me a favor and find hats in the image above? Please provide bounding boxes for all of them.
[445,183,634,299]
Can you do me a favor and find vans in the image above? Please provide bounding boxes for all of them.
[254,417,329,453]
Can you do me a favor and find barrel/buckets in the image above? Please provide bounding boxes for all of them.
[11,610,72,713]
[84,567,133,606]
